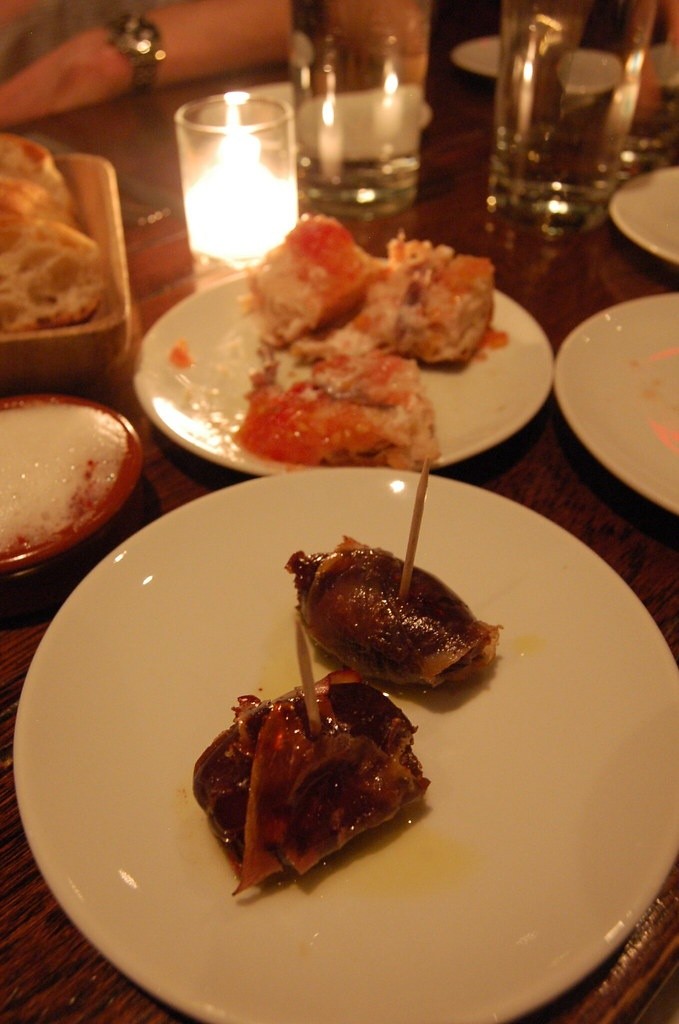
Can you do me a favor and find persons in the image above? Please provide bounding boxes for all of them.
[0,1,430,131]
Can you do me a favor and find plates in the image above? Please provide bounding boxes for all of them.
[133,260,554,472]
[1,155,131,388]
[644,41,679,93]
[1,394,142,581]
[12,468,679,1024]
[452,38,622,95]
[555,293,679,517]
[608,167,679,268]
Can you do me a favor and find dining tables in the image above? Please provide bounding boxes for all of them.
[0,56,679,1023]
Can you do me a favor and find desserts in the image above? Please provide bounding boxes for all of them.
[238,213,498,470]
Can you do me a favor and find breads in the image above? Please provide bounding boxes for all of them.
[0,134,110,333]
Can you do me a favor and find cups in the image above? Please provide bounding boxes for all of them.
[489,1,657,239]
[175,96,297,290]
[291,0,429,219]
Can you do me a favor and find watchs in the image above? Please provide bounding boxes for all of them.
[107,11,162,94]
